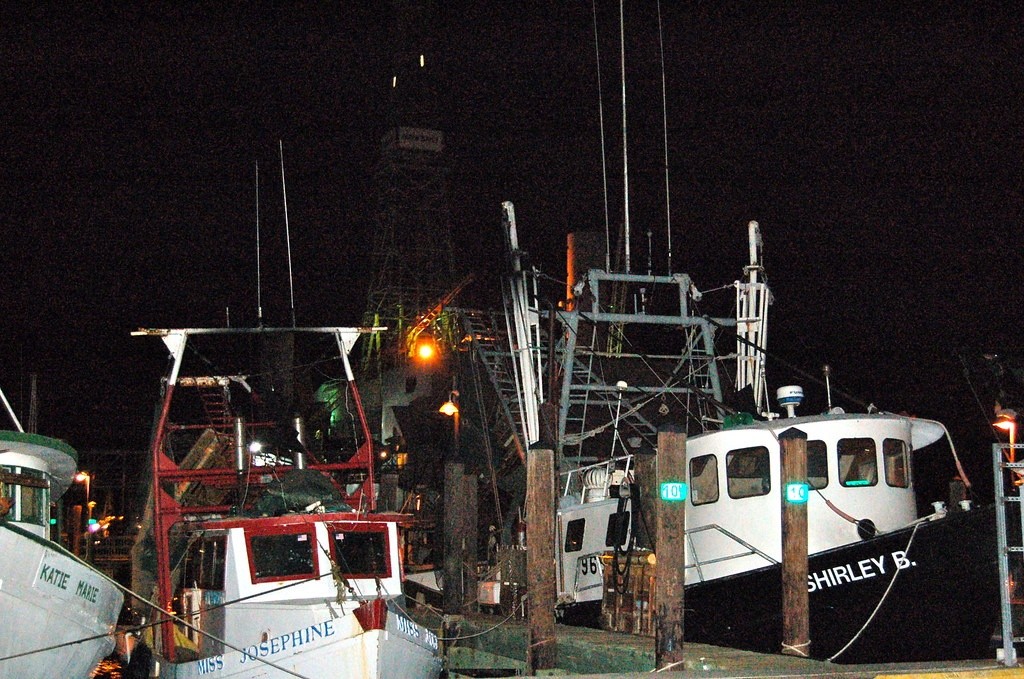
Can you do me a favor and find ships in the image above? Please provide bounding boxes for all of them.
[213,0,1024,650]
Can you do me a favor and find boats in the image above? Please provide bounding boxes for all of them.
[118,326,439,679]
[0,384,125,679]
